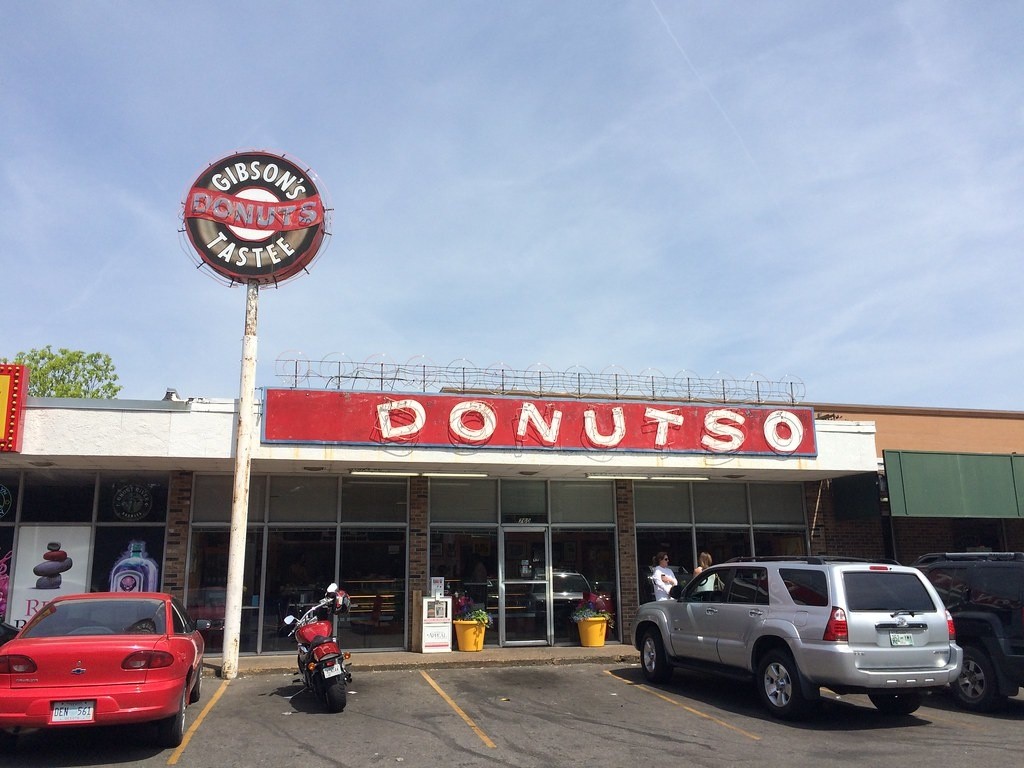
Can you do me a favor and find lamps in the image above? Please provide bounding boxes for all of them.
[349,469,489,477]
[586,473,711,481]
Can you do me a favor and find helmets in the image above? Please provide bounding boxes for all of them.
[333,588,352,616]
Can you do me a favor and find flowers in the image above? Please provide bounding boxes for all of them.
[463,609,494,628]
[569,608,615,629]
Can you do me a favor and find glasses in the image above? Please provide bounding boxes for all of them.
[661,558,669,561]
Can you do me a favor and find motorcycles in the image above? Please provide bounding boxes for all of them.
[284,582,353,712]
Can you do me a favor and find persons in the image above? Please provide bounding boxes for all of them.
[650,552,679,601]
[694,552,715,591]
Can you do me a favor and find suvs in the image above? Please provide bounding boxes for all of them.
[630,553,964,714]
[525,568,591,614]
[906,551,1024,707]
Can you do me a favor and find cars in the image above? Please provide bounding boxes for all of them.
[590,580,616,614]
[186,586,226,632]
[0,591,205,748]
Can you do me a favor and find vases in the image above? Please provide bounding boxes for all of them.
[573,617,610,647]
[453,620,488,651]
